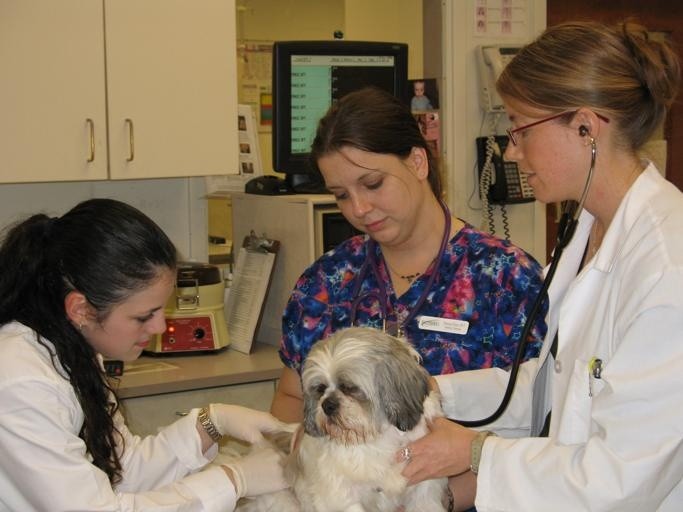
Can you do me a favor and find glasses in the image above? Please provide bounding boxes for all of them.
[504,107,609,145]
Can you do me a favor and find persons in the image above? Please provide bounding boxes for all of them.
[410,81,433,113]
[391,16,682,512]
[268,84,561,511]
[0,195,302,511]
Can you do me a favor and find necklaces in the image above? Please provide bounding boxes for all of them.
[390,269,422,286]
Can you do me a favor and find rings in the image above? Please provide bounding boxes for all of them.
[399,442,412,461]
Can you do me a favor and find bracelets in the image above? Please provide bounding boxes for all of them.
[467,427,497,475]
[195,408,222,445]
[444,483,455,512]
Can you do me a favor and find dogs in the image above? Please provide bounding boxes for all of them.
[238,326,449,512]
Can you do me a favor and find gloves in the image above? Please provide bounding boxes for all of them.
[208,402,301,445]
[222,443,291,501]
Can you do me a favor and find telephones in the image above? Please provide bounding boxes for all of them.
[477,136,536,204]
[476,41,533,114]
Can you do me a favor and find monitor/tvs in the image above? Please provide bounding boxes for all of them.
[271,39,409,195]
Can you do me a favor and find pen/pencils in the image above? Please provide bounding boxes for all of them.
[588,357,603,396]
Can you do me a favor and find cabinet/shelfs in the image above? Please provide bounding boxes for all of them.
[0,0,242,182]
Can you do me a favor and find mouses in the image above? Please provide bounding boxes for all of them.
[245,175,288,195]
